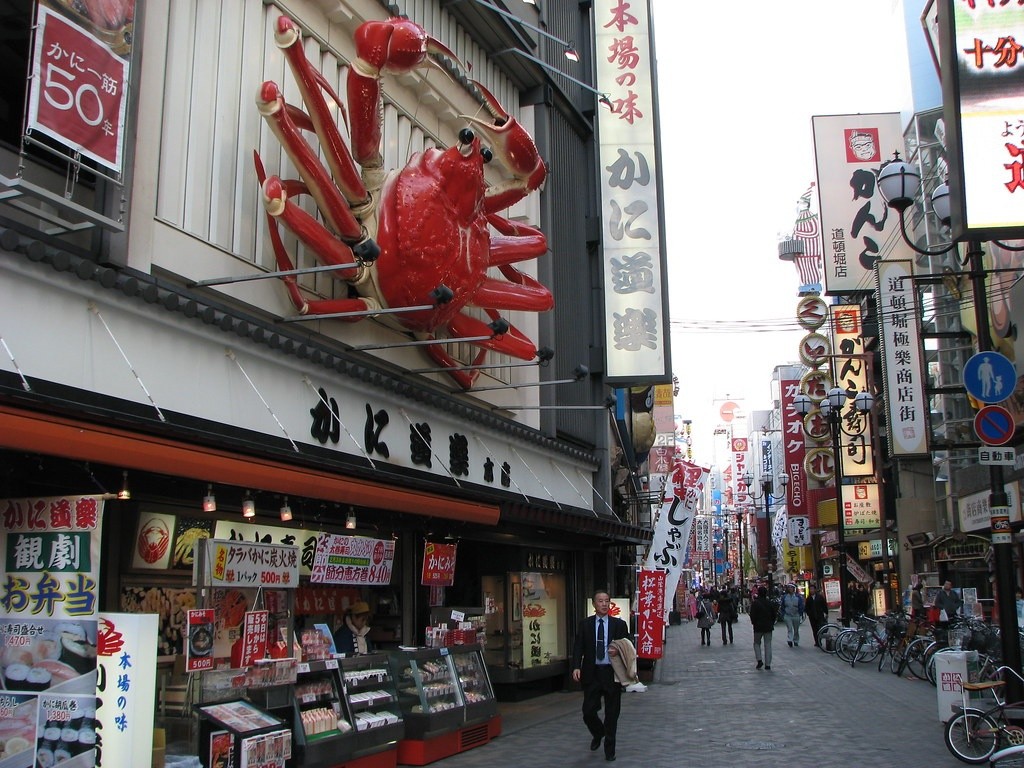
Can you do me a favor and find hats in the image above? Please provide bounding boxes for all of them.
[347,601,370,615]
[785,581,797,587]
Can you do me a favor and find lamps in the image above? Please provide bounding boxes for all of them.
[487,47,614,111]
[405,347,555,375]
[118,470,130,500]
[345,506,356,529]
[475,0,579,62]
[343,318,512,353]
[202,484,217,513]
[280,496,292,522]
[270,282,454,323]
[450,364,590,394]
[180,236,382,289]
[242,489,256,518]
[492,393,618,410]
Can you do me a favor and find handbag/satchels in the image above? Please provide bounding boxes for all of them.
[694,606,705,619]
[940,608,948,621]
[709,617,714,625]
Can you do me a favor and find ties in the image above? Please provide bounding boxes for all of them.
[596,618,605,661]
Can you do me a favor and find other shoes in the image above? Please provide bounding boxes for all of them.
[786,641,793,647]
[722,640,727,644]
[794,642,798,647]
[730,640,733,644]
[756,661,763,668]
[765,665,770,670]
[700,642,706,646]
[707,643,710,646]
[814,644,821,647]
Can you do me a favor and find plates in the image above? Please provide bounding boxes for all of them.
[191,627,210,655]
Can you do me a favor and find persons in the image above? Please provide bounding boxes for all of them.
[332,601,378,658]
[1015,586,1024,629]
[688,581,871,670]
[934,580,961,618]
[912,583,924,621]
[572,591,628,762]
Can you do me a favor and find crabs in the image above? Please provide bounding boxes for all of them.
[255,12,557,388]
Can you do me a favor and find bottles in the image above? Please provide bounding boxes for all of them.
[300,628,331,661]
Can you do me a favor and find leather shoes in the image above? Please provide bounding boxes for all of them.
[605,754,616,762]
[590,730,605,751]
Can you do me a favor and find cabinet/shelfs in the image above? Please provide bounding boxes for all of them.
[279,642,497,768]
